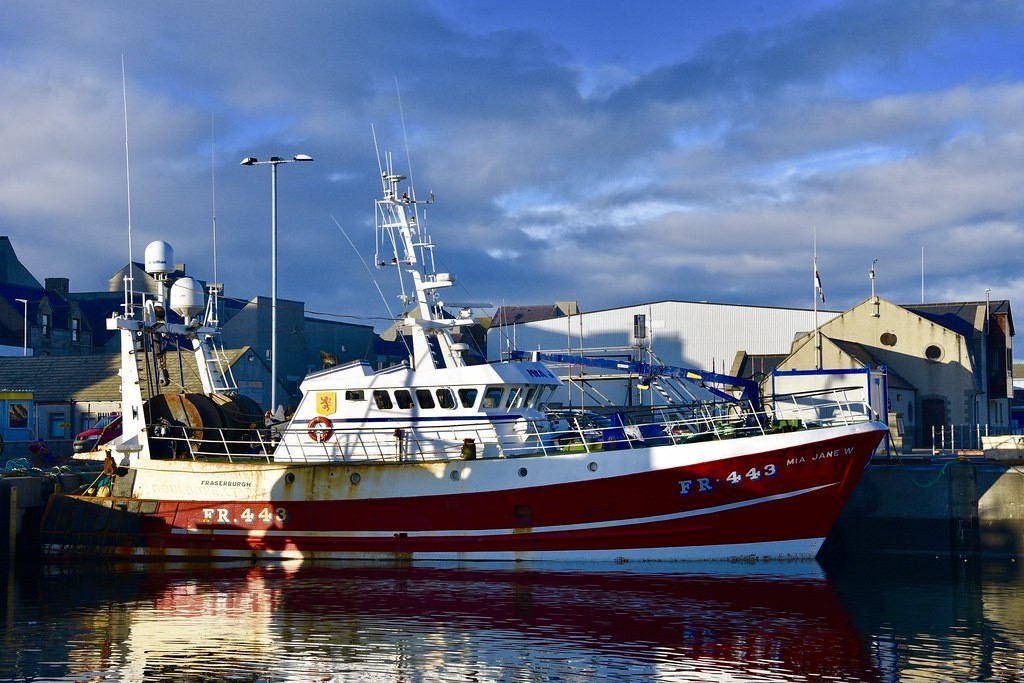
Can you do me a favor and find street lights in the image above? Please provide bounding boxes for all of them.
[14,298,28,355]
[238,152,316,415]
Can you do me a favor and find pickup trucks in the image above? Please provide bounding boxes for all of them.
[71,414,123,454]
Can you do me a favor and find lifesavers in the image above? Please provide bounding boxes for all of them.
[308,416,333,442]
[670,430,683,442]
[265,410,271,419]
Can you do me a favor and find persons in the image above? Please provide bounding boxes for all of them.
[265,405,295,442]
[28,438,48,468]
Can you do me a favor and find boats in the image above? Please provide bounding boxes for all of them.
[34,73,894,585]
